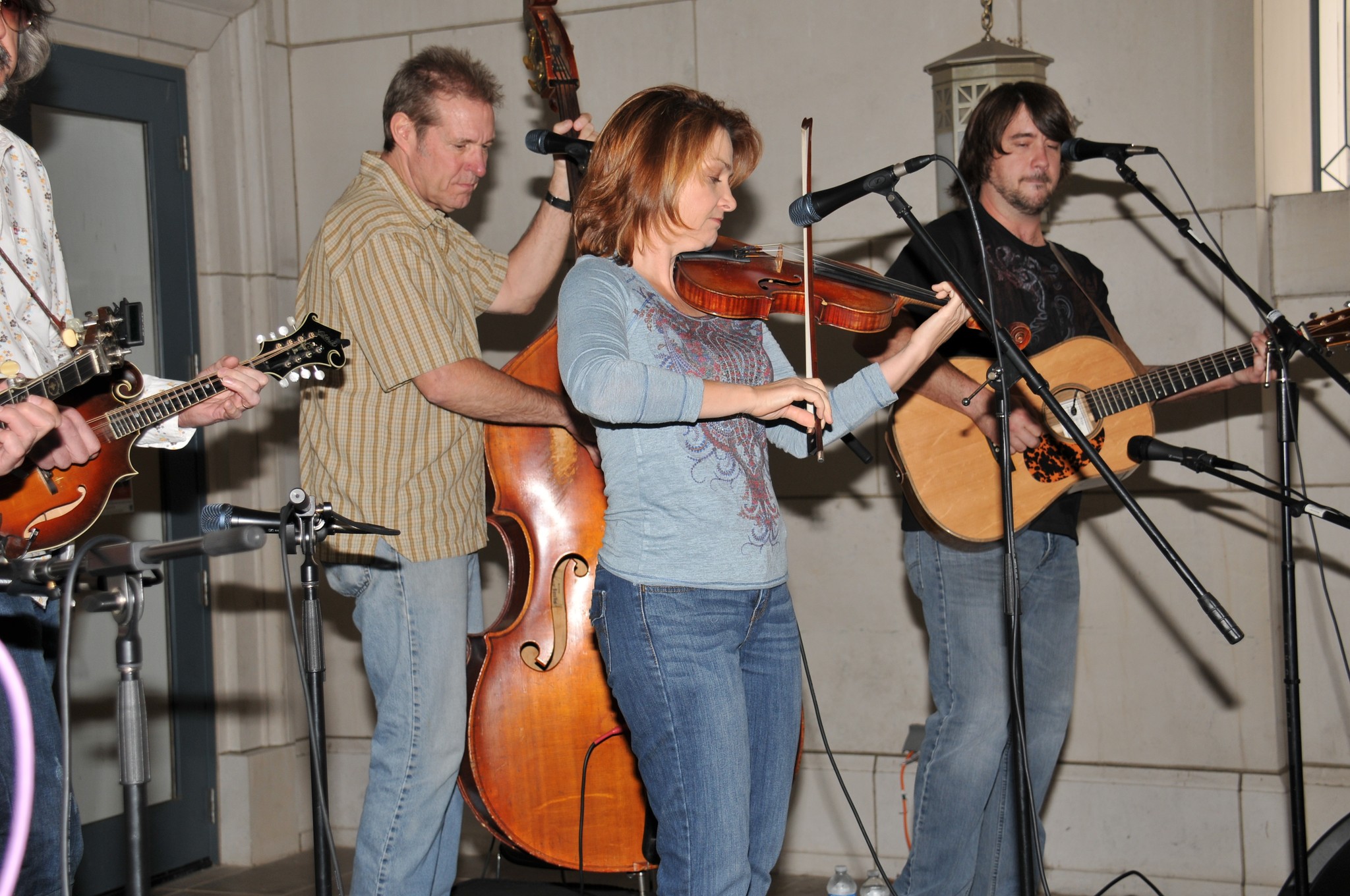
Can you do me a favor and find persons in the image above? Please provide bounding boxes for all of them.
[0,0,269,896]
[294,46,602,896]
[558,84,984,896]
[882,80,1279,896]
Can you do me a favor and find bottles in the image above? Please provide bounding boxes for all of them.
[826,865,857,896]
[859,869,890,896]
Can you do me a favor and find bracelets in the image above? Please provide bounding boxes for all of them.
[544,190,573,213]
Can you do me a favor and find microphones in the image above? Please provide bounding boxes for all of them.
[524,129,596,157]
[1062,136,1158,162]
[200,502,386,534]
[788,153,938,227]
[1127,435,1250,471]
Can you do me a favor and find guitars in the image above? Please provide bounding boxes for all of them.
[0,296,149,437]
[2,308,350,563]
[883,298,1350,555]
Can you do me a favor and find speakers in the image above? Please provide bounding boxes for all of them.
[1278,812,1350,896]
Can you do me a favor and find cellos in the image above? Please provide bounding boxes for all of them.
[457,0,817,893]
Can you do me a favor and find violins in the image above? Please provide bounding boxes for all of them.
[672,232,1033,353]
